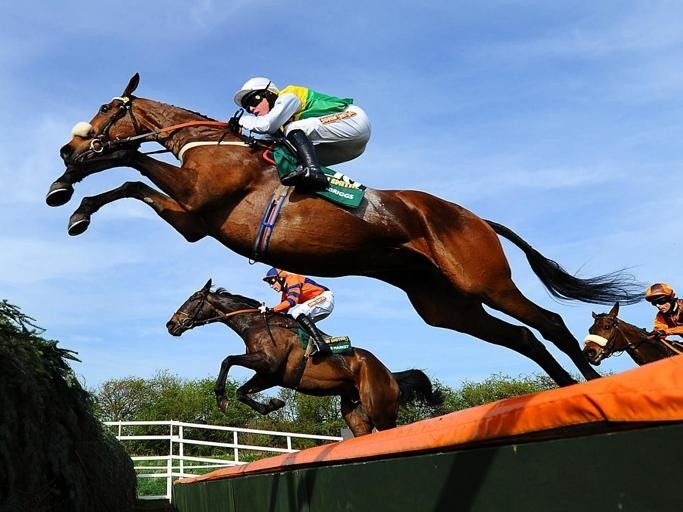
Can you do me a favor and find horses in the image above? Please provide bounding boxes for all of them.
[45,72,647,388]
[165,278,445,438]
[582,301,683,367]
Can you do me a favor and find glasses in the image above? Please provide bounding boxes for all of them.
[242,91,264,114]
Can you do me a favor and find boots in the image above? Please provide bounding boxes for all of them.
[300,316,331,357]
[280,129,327,187]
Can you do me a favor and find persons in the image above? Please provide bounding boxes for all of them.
[233,77,372,195]
[644,283,683,339]
[258,268,335,357]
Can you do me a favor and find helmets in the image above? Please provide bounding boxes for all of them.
[263,268,287,283]
[234,77,280,107]
[645,283,675,302]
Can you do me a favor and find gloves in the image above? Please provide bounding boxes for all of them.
[651,329,666,339]
[259,304,272,315]
[228,116,243,128]
